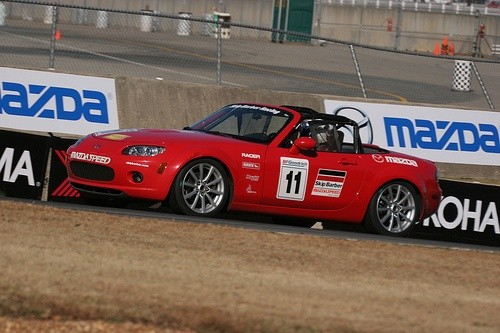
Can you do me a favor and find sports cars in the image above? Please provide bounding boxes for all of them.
[66,101,441,236]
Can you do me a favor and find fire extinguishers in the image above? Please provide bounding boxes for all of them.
[480,24,484,37]
[387,18,392,32]
